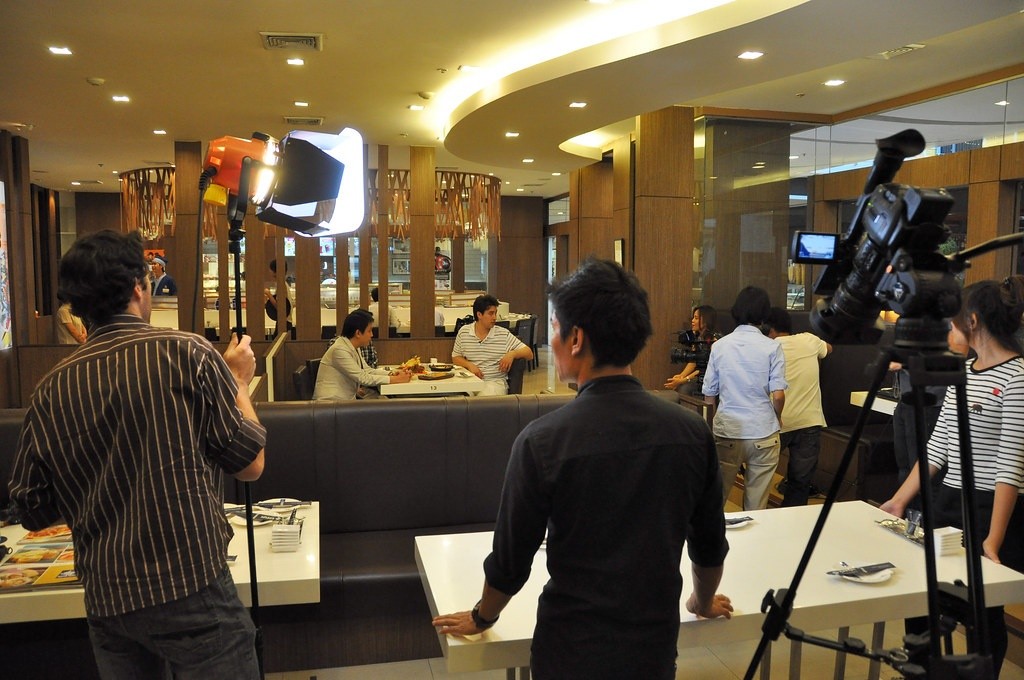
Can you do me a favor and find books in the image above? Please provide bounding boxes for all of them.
[0,522,83,592]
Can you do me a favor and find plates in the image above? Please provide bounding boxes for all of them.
[261,497,300,512]
[419,372,454,379]
[724,513,748,529]
[224,502,239,516]
[843,563,892,583]
[428,363,453,371]
[232,511,279,526]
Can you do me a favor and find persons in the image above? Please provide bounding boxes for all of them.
[761,305,832,507]
[702,283,788,512]
[264,260,293,340]
[214,285,232,310]
[367,288,401,331]
[877,265,1024,680]
[663,303,725,396]
[54,283,87,345]
[451,294,534,397]
[148,257,177,297]
[429,256,733,680]
[7,226,268,680]
[311,308,412,400]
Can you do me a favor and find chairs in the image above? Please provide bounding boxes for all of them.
[205,316,542,399]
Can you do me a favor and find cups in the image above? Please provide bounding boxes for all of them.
[430,357,438,364]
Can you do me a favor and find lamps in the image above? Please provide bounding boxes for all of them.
[201,126,365,238]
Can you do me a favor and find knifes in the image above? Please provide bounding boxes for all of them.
[826,562,895,576]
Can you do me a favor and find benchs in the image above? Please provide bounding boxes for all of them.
[0,388,680,680]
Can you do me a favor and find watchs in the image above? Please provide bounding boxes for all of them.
[470,599,501,630]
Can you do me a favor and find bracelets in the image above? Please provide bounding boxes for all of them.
[686,376,690,383]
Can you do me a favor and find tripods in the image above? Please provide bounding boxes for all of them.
[742,231,1024,680]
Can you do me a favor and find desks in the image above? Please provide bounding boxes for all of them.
[0,496,319,626]
[378,362,486,401]
[414,500,1024,680]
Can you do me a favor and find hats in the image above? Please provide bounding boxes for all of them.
[152,258,166,272]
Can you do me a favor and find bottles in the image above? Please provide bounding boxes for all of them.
[788,259,806,285]
